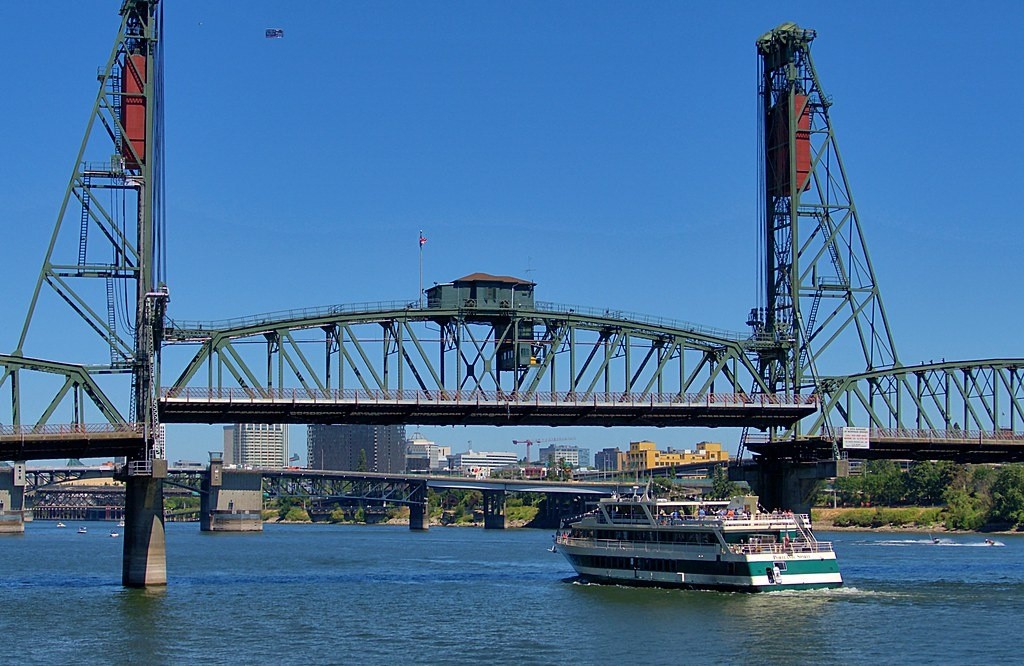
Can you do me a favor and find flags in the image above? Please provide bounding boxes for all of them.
[419,235,427,245]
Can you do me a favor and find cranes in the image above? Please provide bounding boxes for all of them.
[513,437,577,466]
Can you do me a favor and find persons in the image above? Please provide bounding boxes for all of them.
[557,530,567,541]
[738,538,747,553]
[657,508,794,525]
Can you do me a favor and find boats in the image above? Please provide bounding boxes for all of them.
[110,530,119,537]
[116,521,125,527]
[552,475,845,592]
[78,524,86,533]
[56,522,66,528]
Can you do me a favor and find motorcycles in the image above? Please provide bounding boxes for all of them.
[933,538,939,543]
[986,539,994,545]
